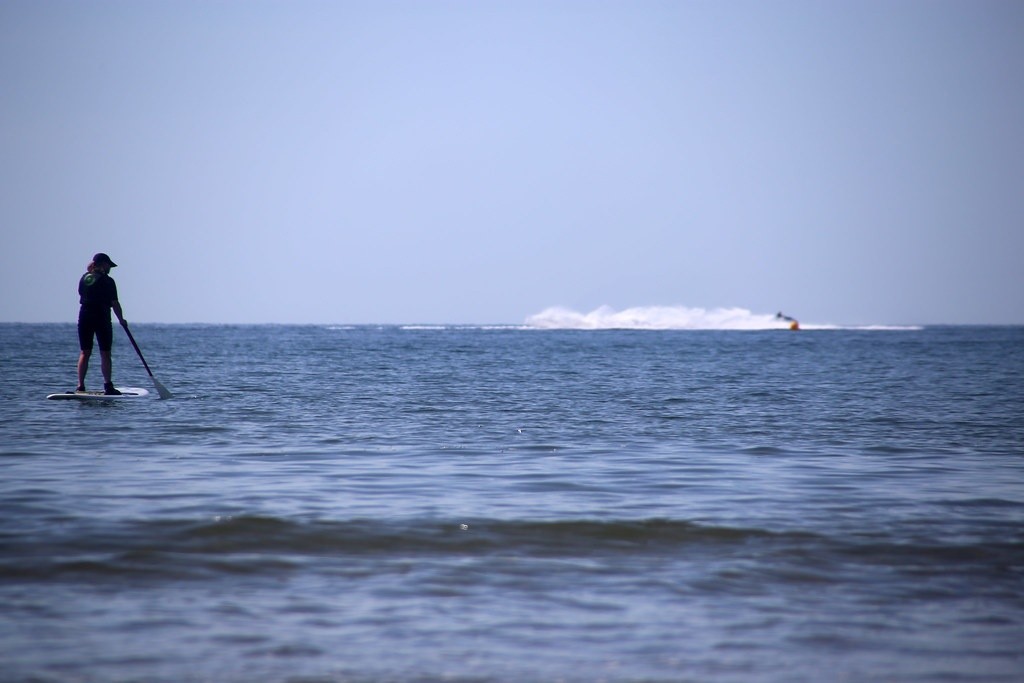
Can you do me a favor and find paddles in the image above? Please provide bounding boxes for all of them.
[123,325,173,400]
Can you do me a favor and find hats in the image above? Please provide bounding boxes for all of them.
[93,252,118,268]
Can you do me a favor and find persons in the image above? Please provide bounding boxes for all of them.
[76,253,128,393]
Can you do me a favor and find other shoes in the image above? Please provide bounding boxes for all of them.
[76,387,85,394]
[104,383,122,395]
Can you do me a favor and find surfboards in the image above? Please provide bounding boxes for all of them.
[46,387,149,400]
